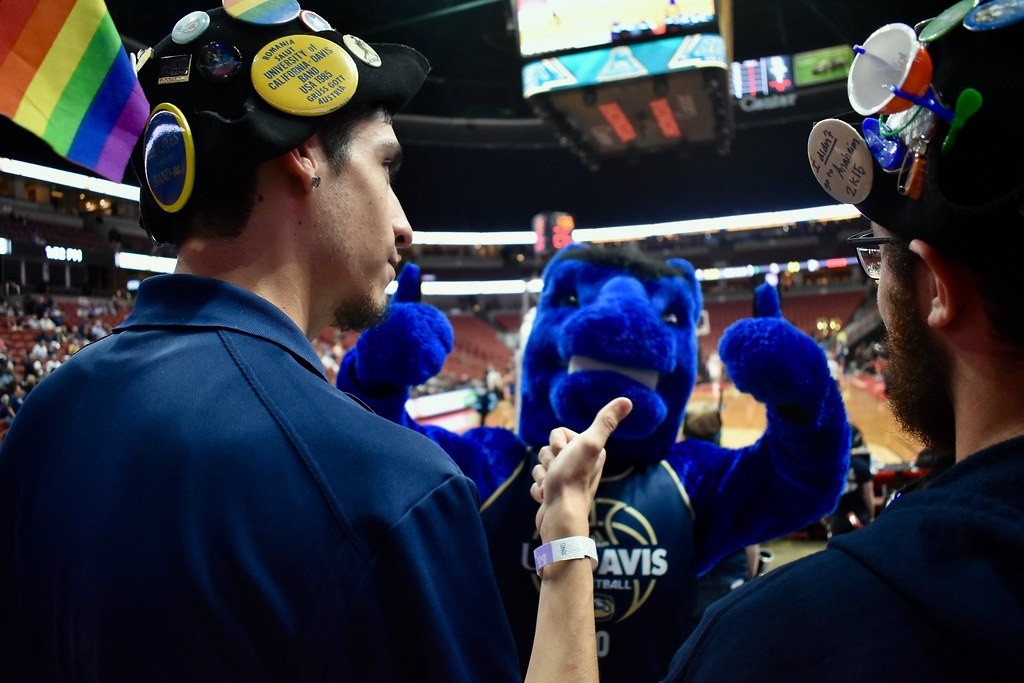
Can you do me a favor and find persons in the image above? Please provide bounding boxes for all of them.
[0,0,1024,683]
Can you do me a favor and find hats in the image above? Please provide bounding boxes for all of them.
[809,18,1023,276]
[119,5,433,247]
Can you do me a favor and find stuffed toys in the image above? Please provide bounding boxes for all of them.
[337,244,851,683]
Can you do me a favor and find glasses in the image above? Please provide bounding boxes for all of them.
[847,229,892,280]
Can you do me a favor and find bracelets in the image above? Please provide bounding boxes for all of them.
[534,536,599,577]
